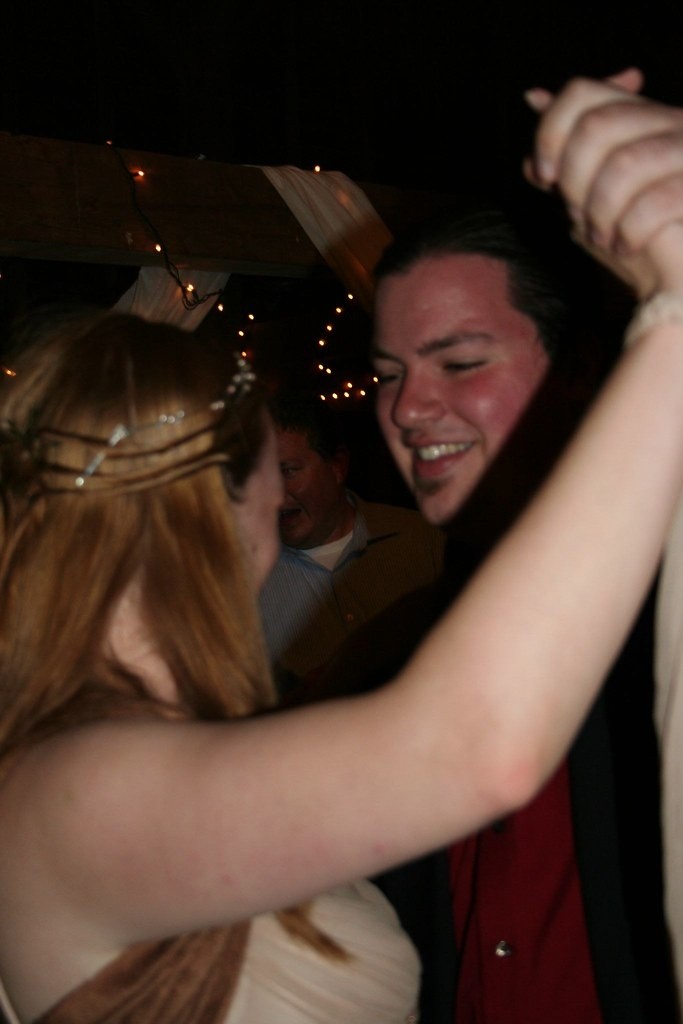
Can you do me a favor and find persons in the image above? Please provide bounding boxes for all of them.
[0,59,682,1022]
